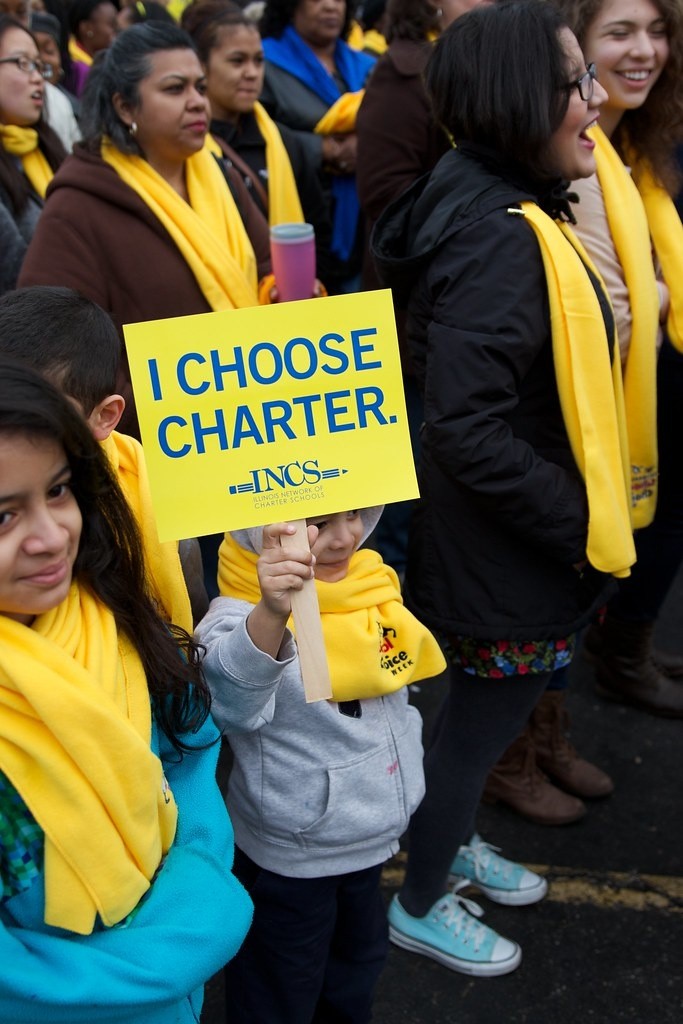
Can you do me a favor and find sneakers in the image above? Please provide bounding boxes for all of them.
[386,880,522,976]
[447,834,549,906]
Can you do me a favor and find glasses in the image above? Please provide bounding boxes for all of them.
[0,56,54,77]
[556,62,596,101]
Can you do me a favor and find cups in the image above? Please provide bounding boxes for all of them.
[269,224,318,303]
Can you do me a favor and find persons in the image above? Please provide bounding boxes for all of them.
[0,361,253,1024]
[189,504,449,1024]
[0,0,683,888]
[362,0,637,978]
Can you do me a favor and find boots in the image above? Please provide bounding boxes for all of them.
[581,621,683,676]
[483,724,586,826]
[595,619,683,719]
[525,689,615,799]
